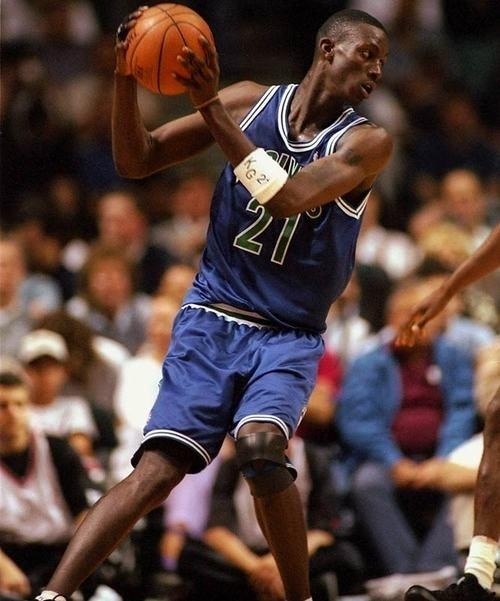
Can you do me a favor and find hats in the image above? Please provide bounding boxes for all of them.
[18,328,70,369]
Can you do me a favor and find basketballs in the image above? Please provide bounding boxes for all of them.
[125,4,215,96]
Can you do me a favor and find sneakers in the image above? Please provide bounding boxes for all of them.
[403,572,497,601]
